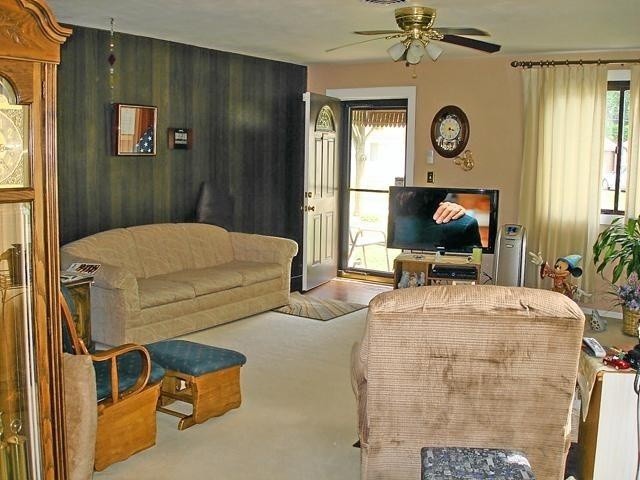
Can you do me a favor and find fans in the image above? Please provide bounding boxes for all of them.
[325,6,501,56]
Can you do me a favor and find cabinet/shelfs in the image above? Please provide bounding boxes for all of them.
[394,259,481,290]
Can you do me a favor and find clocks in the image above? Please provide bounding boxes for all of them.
[429,104,472,159]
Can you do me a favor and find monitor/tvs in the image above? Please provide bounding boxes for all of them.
[386,186,499,254]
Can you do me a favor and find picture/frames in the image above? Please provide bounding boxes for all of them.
[115,102,157,158]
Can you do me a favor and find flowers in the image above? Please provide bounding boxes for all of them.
[619,273,640,313]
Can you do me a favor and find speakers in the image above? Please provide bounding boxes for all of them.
[493,224,528,287]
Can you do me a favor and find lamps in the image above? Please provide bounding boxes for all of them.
[386,36,445,65]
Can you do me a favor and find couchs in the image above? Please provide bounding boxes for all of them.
[60,222,301,345]
[349,285,586,480]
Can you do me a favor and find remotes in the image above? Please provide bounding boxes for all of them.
[582,337,606,357]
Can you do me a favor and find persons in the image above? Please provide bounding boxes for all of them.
[391,190,484,250]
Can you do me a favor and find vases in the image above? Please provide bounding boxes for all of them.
[622,306,640,337]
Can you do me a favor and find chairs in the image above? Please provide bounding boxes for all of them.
[61,281,167,472]
[349,221,390,272]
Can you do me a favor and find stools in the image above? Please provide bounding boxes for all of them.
[145,339,247,431]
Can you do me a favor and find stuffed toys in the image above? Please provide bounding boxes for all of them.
[525,248,583,302]
[397,270,426,290]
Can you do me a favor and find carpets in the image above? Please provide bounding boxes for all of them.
[269,289,372,323]
